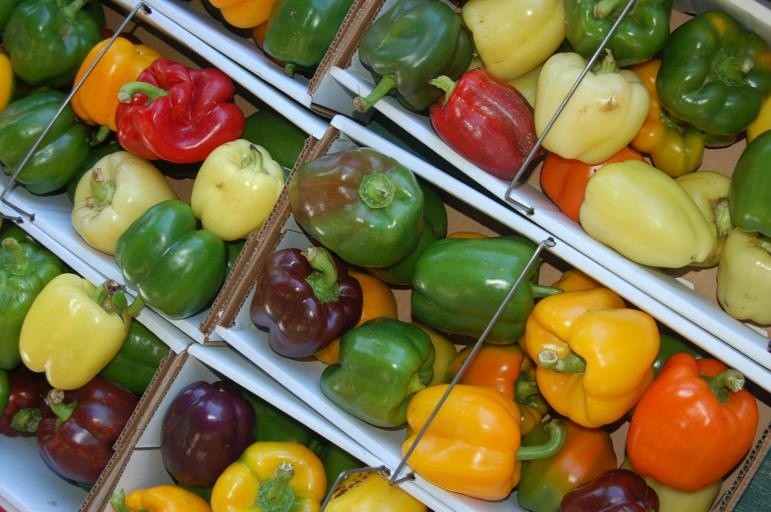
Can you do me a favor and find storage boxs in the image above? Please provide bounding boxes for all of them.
[0,0,771,512]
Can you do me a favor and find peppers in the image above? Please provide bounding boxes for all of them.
[2,1,770,509]
